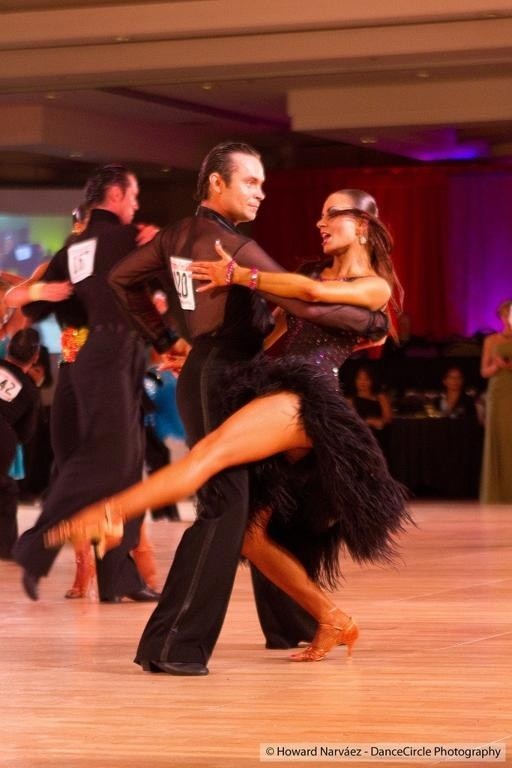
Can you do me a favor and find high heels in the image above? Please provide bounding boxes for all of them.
[288,606,359,663]
[44,496,125,562]
[133,544,159,590]
[65,559,96,599]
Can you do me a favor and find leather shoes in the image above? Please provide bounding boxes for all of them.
[100,585,162,602]
[23,569,40,601]
[140,655,209,676]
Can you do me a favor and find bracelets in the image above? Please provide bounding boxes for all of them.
[248,265,257,291]
[224,258,234,289]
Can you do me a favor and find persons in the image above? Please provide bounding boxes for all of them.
[340,365,391,431]
[479,303,511,506]
[106,140,333,679]
[2,160,183,609]
[427,364,472,417]
[36,185,399,666]
